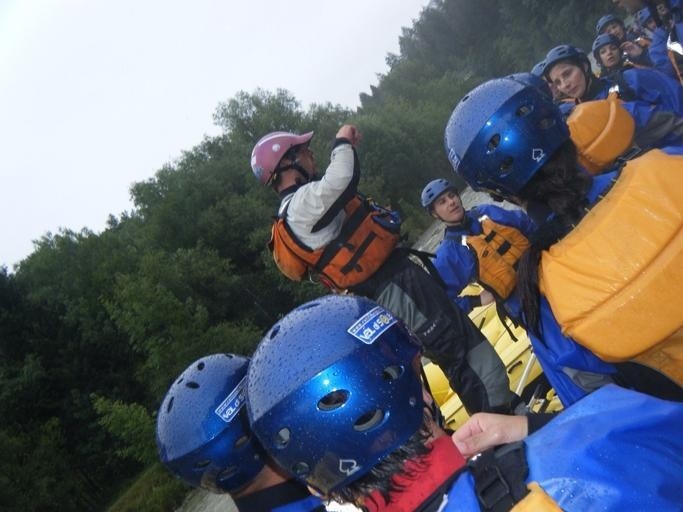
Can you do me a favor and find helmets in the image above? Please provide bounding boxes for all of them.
[155,353,265,494]
[444,77,569,198]
[505,2,681,102]
[421,178,456,211]
[245,294,424,496]
[250,130,314,189]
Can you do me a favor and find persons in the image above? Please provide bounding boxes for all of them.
[150,349,325,509]
[248,122,541,417]
[243,293,681,511]
[416,0,681,405]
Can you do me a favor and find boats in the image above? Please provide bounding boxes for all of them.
[421,289,548,436]
